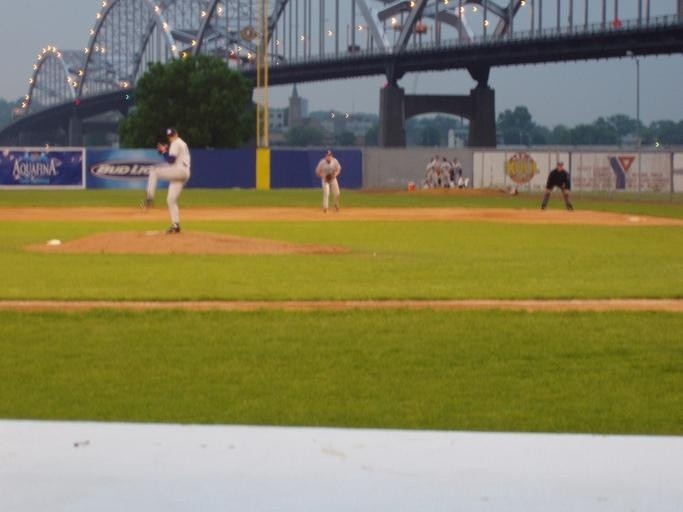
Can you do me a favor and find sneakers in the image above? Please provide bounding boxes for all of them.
[165,221,181,236]
[138,196,155,209]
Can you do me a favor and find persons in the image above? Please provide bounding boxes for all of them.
[539,160,573,211]
[421,155,469,189]
[143,128,192,235]
[315,148,340,212]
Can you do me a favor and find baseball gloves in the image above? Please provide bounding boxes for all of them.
[157,142,168,155]
[325,174,333,183]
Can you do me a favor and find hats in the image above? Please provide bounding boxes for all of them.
[326,150,333,156]
[165,127,176,137]
